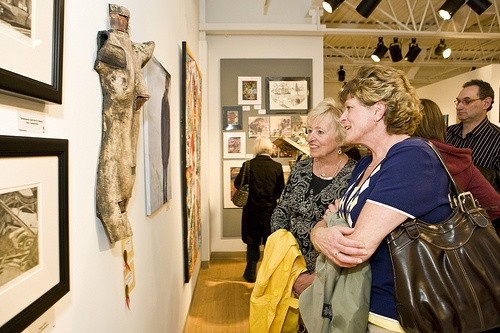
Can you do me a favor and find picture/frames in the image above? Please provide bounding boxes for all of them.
[0,0,65,104]
[0,135,70,333]
[223,76,311,209]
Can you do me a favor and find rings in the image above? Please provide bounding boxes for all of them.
[334,252,340,258]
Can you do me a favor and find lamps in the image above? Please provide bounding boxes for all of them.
[322,0,493,82]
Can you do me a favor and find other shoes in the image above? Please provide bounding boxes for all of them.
[243,274,257,283]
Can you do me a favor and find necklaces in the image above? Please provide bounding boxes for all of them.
[317,153,344,176]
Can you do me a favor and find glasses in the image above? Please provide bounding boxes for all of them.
[453,98,483,105]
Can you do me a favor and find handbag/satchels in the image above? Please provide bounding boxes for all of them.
[386,141,500,333]
[232,161,250,207]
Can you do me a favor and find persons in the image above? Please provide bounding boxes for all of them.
[409,98,500,241]
[234,137,285,283]
[298,63,458,333]
[445,79,500,170]
[270,98,360,333]
